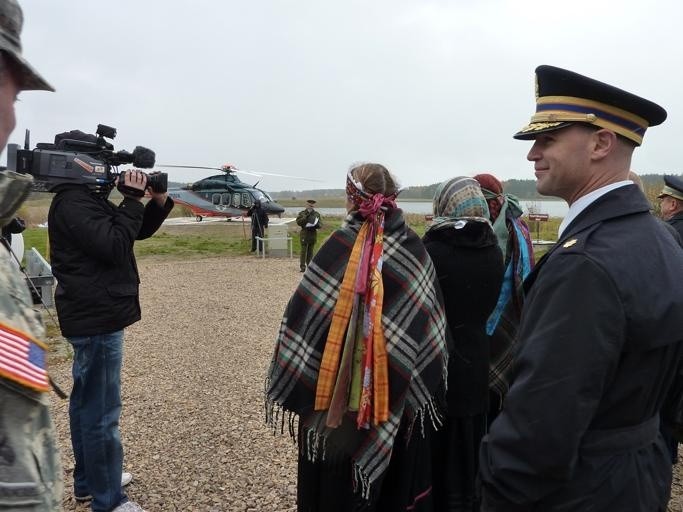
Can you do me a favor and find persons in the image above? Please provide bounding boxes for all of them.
[295,199,324,272]
[262,164,460,510]
[405,175,521,510]
[47,131,177,512]
[248,199,270,254]
[627,171,683,246]
[474,174,535,342]
[1,0,68,512]
[479,64,683,512]
[657,175,682,232]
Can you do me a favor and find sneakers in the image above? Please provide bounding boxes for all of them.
[75,472,132,503]
[113,500,144,512]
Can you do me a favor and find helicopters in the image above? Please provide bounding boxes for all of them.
[152,162,295,221]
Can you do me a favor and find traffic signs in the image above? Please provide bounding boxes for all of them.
[529,214,548,222]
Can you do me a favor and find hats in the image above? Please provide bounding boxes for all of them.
[306,200,315,207]
[657,174,682,200]
[514,64,666,146]
[1,0,56,92]
